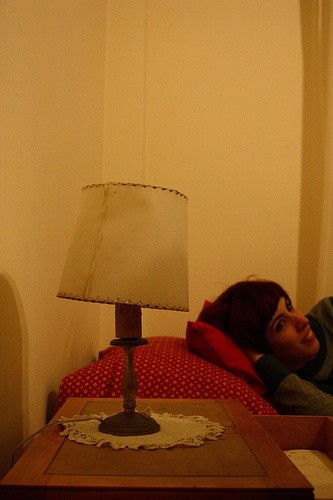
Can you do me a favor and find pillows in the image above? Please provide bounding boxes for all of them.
[186,299,269,393]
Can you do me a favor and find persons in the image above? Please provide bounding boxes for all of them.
[200,277,333,414]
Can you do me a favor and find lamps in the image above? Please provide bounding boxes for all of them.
[56,183,191,436]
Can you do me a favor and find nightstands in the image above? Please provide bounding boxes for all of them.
[1,397,332,500]
